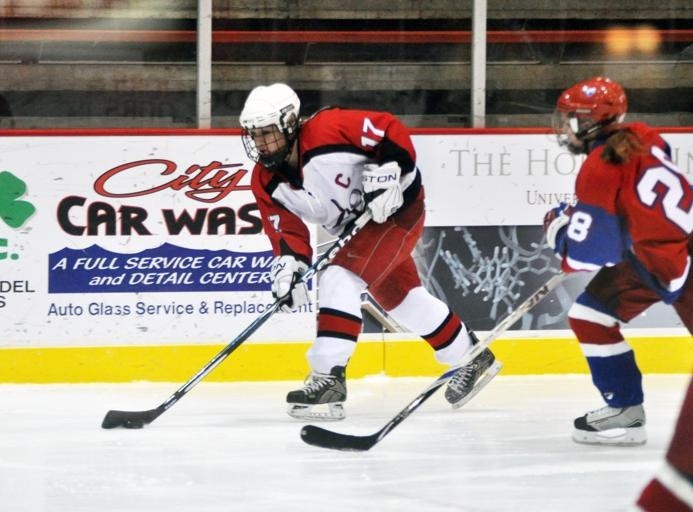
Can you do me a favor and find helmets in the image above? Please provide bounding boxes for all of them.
[239,83,301,168]
[551,78,627,155]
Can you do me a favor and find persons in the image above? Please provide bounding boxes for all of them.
[543,76,693,447]
[238,83,501,422]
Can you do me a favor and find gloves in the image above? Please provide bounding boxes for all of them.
[270,255,311,310]
[362,162,404,223]
[544,202,572,260]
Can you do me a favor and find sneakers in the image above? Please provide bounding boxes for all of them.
[573,403,645,431]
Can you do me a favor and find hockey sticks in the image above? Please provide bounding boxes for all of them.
[102,211,371,428]
[301,270,574,451]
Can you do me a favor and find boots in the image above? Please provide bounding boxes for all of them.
[287,365,346,404]
[445,323,495,403]
